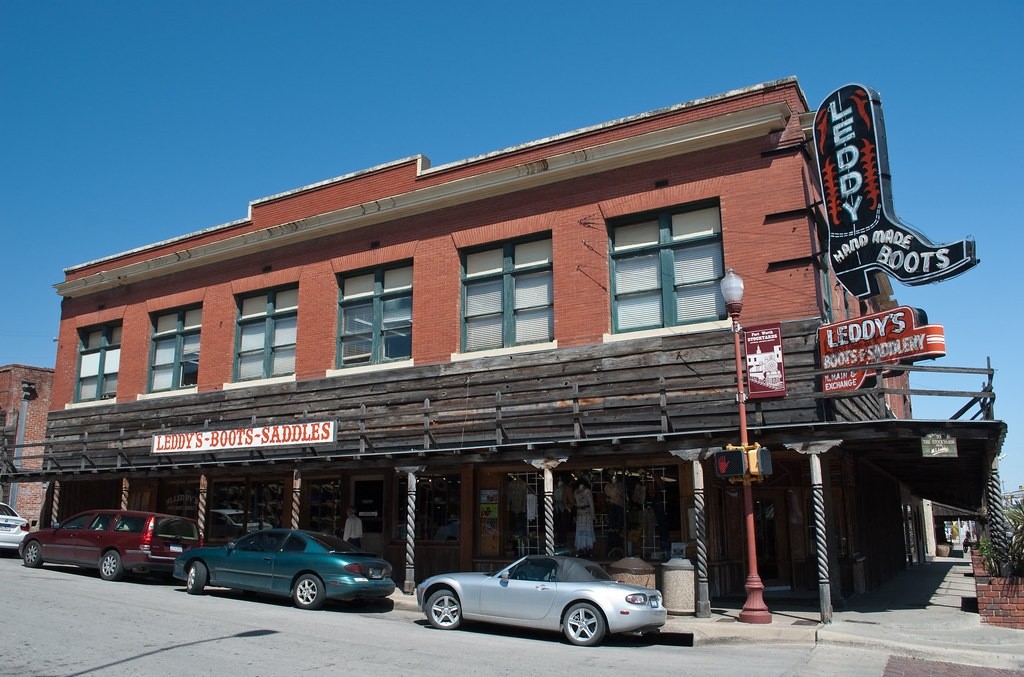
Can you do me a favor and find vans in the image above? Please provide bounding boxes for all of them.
[18,509,202,583]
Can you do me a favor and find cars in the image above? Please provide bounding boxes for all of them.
[0,502,30,550]
[208,508,275,533]
[173,530,399,608]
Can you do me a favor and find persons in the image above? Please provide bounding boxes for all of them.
[574,482,597,559]
[553,479,575,551]
[343,503,363,549]
[603,474,630,561]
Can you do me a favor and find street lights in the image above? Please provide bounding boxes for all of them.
[720,267,774,625]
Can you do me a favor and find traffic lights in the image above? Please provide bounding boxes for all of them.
[715,450,744,476]
[749,448,771,475]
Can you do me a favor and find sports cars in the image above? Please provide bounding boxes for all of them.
[414,555,668,646]
